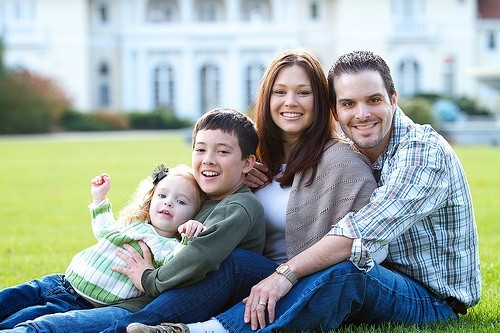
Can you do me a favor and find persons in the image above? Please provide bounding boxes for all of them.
[126,49,484,333]
[0,164,206,333]
[97,47,376,333]
[12,106,266,333]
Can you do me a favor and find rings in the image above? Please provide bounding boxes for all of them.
[258,301,266,306]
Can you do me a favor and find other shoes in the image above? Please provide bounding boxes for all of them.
[126,322,190,333]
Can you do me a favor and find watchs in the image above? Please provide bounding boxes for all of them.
[275,264,298,286]
